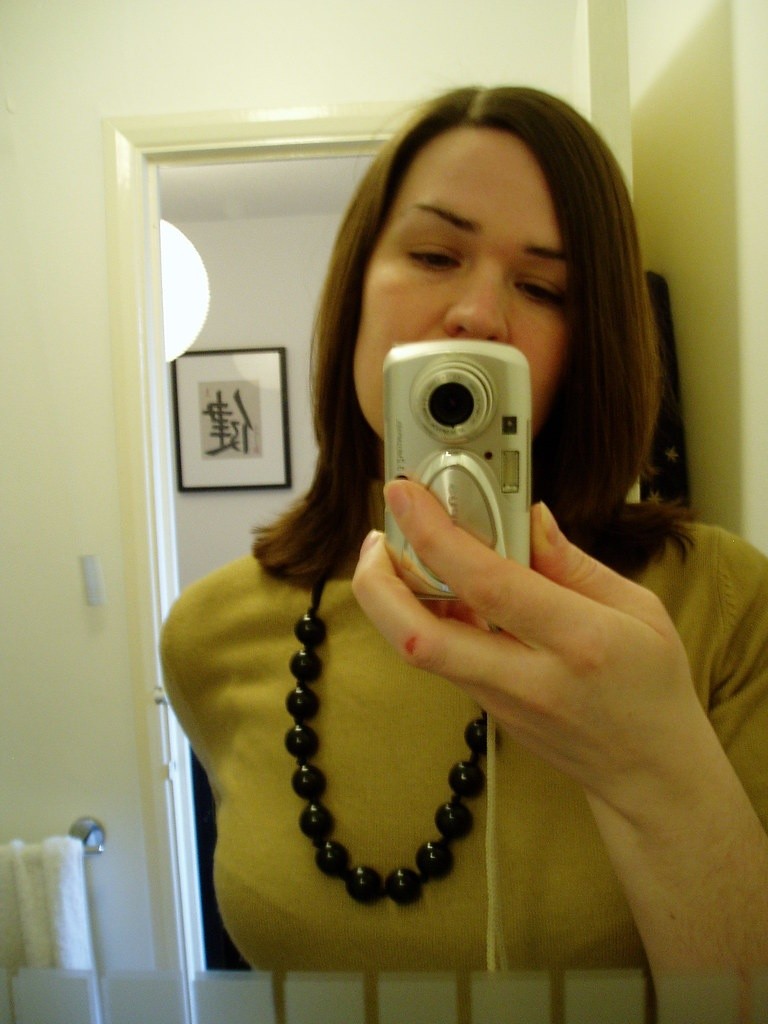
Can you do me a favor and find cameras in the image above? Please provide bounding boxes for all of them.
[382,338,532,599]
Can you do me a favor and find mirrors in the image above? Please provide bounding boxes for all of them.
[1,0,768,1024]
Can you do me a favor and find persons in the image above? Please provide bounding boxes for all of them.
[158,83,768,1024]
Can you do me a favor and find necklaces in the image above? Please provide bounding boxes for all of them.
[285,566,503,909]
